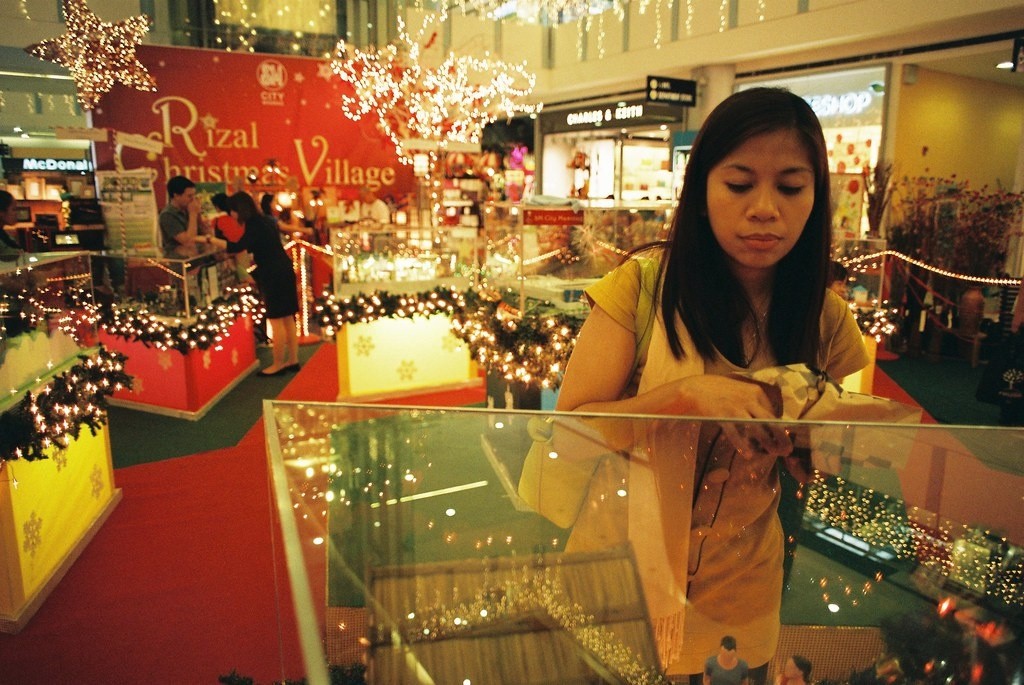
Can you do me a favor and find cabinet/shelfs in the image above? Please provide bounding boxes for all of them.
[1,202,1023,684]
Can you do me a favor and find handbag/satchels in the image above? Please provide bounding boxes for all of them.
[518,256,661,530]
[215,216,228,241]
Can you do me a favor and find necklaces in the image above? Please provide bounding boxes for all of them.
[742,300,767,339]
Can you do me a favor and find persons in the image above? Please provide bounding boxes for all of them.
[357,184,390,225]
[703,635,749,684]
[0,189,25,255]
[159,175,316,378]
[774,654,813,685]
[551,86,870,685]
[827,260,848,299]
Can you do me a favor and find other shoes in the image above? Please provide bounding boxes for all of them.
[256,363,300,377]
